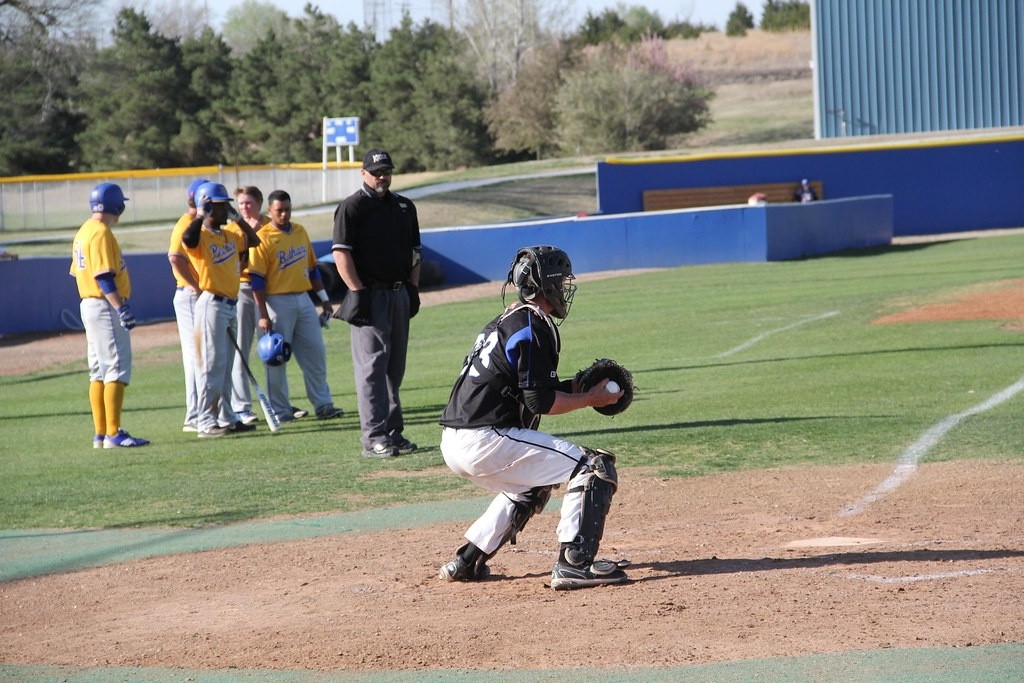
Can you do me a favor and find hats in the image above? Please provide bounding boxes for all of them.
[189,179,208,199]
[801,178,808,185]
[363,149,395,172]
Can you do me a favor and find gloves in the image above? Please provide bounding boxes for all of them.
[197,195,213,220]
[227,204,242,222]
[117,304,136,331]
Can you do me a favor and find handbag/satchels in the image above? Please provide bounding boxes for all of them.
[332,282,373,326]
[406,282,421,318]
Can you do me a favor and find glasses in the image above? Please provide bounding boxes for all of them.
[370,167,393,177]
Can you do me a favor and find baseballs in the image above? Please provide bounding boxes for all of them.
[606,381,620,394]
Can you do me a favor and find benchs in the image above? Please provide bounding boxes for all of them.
[643,181,822,211]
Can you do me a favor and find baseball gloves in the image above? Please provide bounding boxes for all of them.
[577,358,635,417]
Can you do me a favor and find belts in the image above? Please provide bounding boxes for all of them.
[214,295,238,305]
[366,282,407,291]
[177,287,184,291]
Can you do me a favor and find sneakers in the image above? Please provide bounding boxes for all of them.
[439,556,489,582]
[317,408,343,419]
[551,560,627,589]
[220,421,256,433]
[233,411,258,424]
[291,407,307,418]
[92,435,105,448]
[394,437,418,454]
[198,425,230,438]
[183,424,199,432]
[103,428,150,448]
[276,416,296,423]
[362,441,400,458]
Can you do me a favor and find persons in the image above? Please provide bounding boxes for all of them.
[331,149,423,459]
[438,245,634,589]
[69,182,150,448]
[231,186,309,423]
[167,179,210,431]
[248,190,343,419]
[796,179,817,202]
[179,182,261,437]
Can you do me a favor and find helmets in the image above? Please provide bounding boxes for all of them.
[508,245,577,327]
[194,182,234,208]
[89,182,129,215]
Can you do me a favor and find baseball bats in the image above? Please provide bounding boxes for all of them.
[226,325,282,435]
